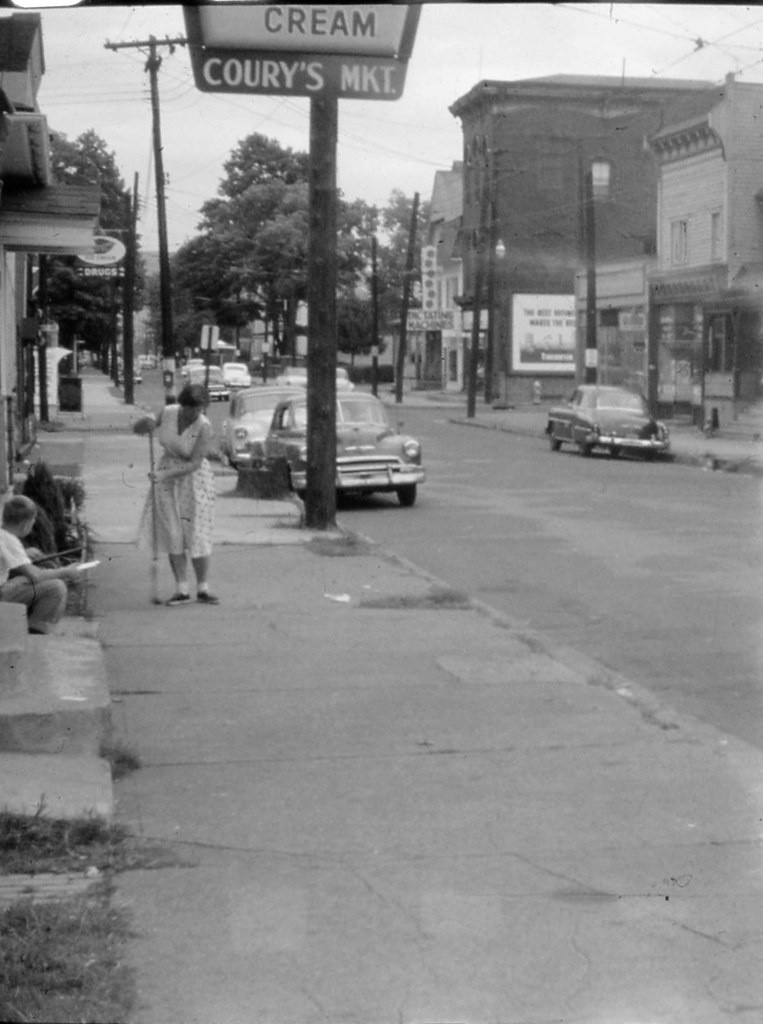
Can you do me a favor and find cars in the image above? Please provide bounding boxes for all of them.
[182,359,204,377]
[140,356,155,369]
[222,361,251,388]
[266,394,426,506]
[275,367,309,390]
[224,386,303,475]
[544,385,670,464]
[188,365,231,400]
[118,365,142,385]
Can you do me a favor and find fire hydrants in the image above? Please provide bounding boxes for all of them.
[534,384,541,403]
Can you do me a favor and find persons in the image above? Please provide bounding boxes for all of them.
[134,384,220,606]
[0,495,89,635]
[704,408,719,439]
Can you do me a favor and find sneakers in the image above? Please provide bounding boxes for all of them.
[196,590,220,605]
[166,592,192,605]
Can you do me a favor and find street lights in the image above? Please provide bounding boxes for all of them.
[74,341,85,379]
[484,239,506,407]
